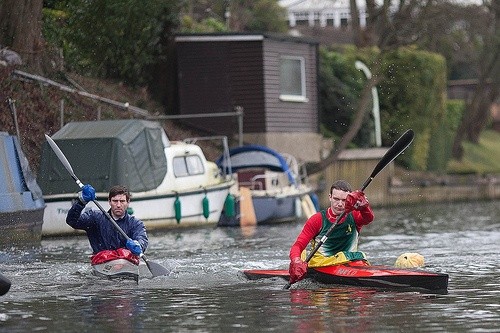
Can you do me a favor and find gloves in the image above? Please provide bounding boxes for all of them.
[289,257,308,284]
[126,238,143,256]
[345,191,371,215]
[78,183,97,204]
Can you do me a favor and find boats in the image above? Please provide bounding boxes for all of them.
[211,143,317,225]
[245,266,449,299]
[40,118,235,236]
[92,259,140,283]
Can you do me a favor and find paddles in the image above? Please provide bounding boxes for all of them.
[283,128,414,290]
[45,134,171,279]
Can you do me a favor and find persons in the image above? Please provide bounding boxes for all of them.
[289,180,374,280]
[66,185,148,255]
